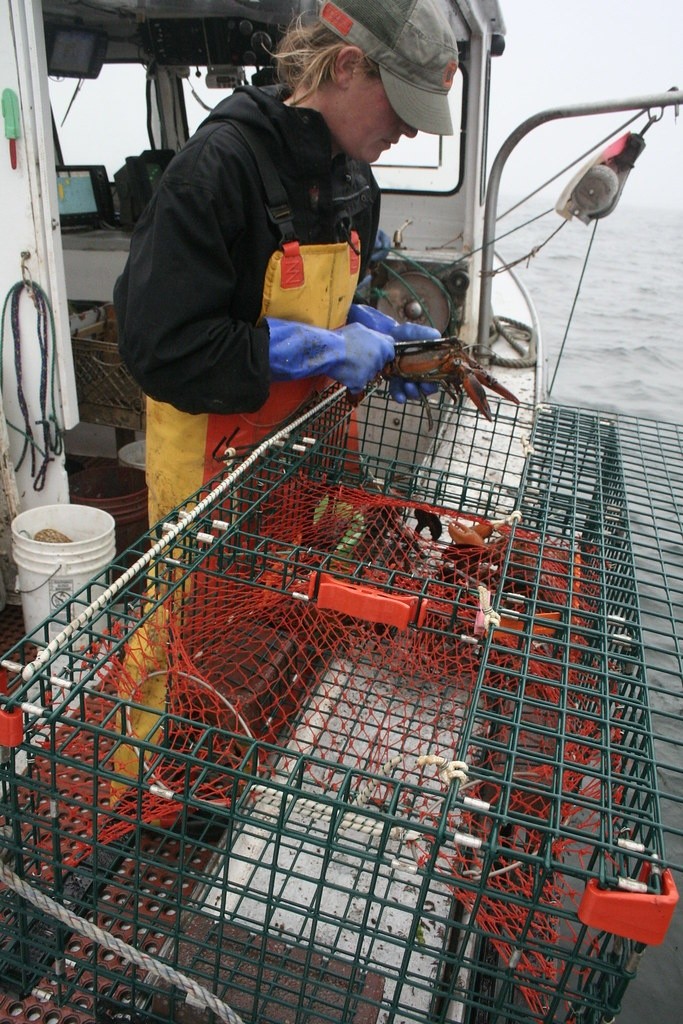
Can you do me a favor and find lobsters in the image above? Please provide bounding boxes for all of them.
[346,348,520,430]
[440,519,556,616]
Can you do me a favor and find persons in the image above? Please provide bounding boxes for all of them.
[110,0,460,831]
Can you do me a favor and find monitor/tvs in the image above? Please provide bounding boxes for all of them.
[42,25,108,79]
[55,165,116,234]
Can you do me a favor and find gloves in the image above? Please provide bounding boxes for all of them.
[261,315,394,395]
[346,303,442,404]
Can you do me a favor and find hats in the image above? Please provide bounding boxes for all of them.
[318,0,459,136]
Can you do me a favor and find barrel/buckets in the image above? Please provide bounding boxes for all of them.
[5,436,148,653]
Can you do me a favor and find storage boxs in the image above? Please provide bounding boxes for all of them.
[70,318,146,434]
